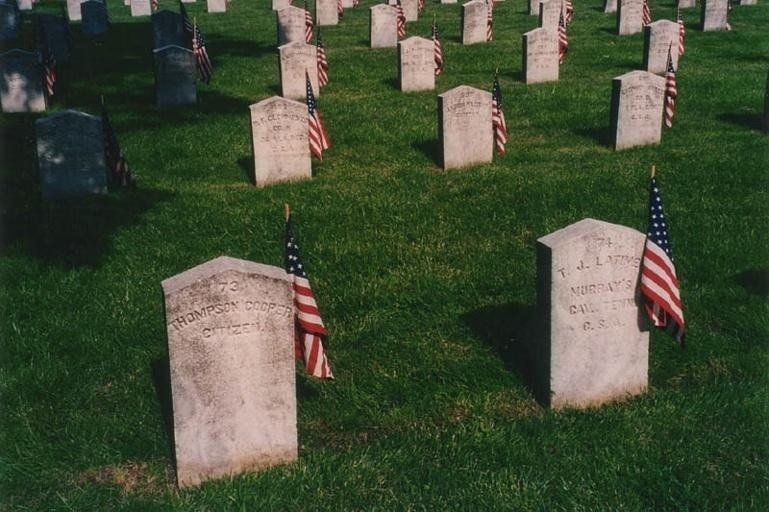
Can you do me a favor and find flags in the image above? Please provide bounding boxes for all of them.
[643,0,686,56]
[337,1,494,42]
[557,1,574,61]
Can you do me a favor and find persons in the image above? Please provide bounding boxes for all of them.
[317,24,328,87]
[492,71,508,155]
[181,1,193,32]
[432,18,443,76]
[305,1,313,43]
[37,13,57,98]
[101,102,133,194]
[151,0,159,10]
[192,22,214,85]
[641,178,685,347]
[306,72,332,162]
[285,217,335,379]
[665,49,678,129]
